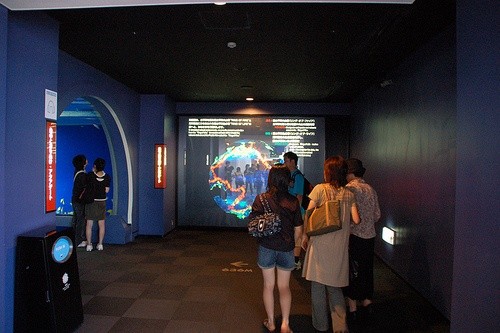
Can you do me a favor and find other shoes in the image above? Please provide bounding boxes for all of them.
[96,243,103,251]
[78,241,87,248]
[346,310,359,319]
[360,302,376,313]
[294,260,301,270]
[86,244,93,252]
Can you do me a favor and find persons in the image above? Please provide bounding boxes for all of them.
[224,153,283,196]
[250,151,381,333]
[72,155,110,252]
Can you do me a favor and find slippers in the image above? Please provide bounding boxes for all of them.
[264,319,276,332]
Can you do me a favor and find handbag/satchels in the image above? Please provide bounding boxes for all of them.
[305,183,342,235]
[247,192,282,238]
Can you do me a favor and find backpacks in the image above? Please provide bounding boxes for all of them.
[290,170,314,210]
